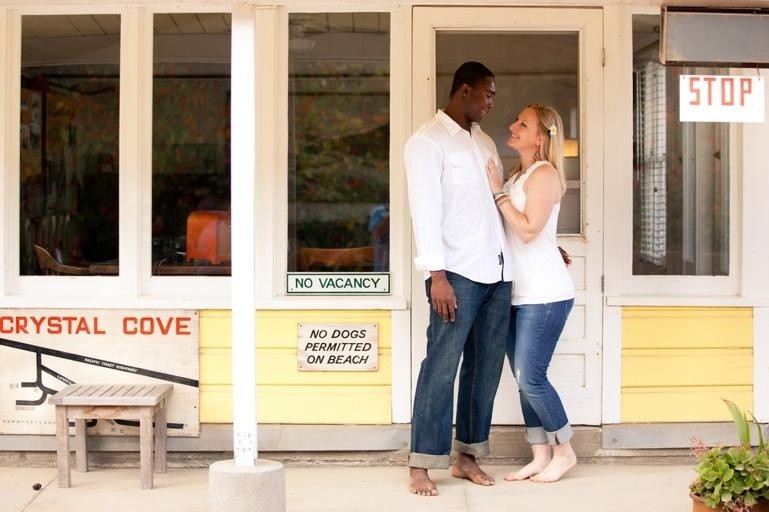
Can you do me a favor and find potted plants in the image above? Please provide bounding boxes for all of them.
[687,399,769,512]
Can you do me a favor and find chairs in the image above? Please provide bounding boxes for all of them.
[295,245,389,272]
[33,244,89,275]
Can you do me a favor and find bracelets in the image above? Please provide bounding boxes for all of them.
[493,191,511,205]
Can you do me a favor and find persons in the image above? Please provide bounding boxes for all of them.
[405,60,572,496]
[485,101,578,484]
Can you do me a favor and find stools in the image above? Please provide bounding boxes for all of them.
[48,384,173,490]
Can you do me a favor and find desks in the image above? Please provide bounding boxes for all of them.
[89,260,230,276]
